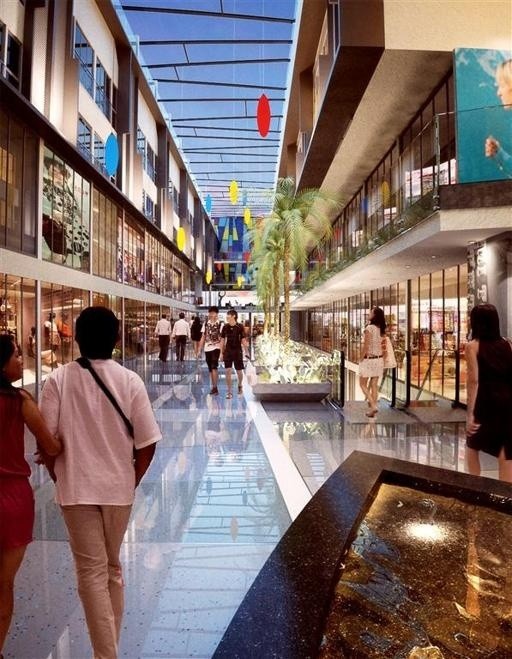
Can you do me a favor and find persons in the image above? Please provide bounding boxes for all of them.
[220,311,246,399]
[0,328,63,659]
[44,157,90,264]
[465,303,512,485]
[484,60,512,180]
[358,307,387,418]
[196,306,226,395]
[26,310,72,367]
[156,312,248,363]
[37,305,163,659]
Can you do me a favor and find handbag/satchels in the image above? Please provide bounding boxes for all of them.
[382,336,397,369]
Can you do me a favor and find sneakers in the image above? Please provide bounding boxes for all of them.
[226,392,234,399]
[237,385,244,395]
[209,386,219,395]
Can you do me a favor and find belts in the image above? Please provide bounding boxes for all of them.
[363,355,384,360]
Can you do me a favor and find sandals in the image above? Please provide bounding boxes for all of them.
[366,406,378,420]
